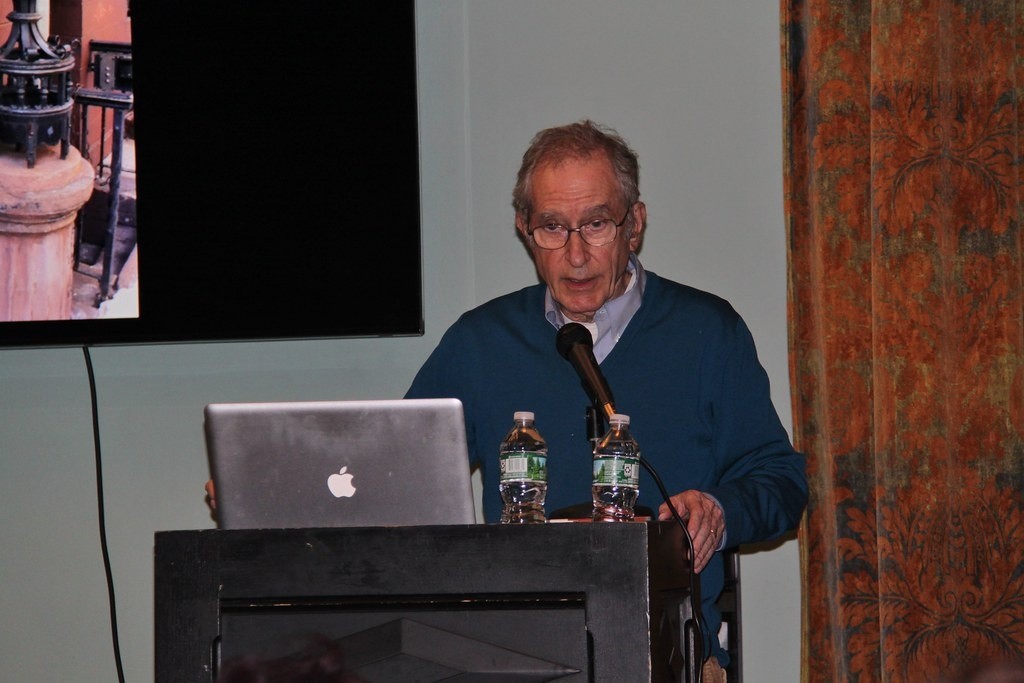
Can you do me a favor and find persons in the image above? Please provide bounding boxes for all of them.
[204,119,809,683]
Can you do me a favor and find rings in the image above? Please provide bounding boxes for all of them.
[711,530,717,540]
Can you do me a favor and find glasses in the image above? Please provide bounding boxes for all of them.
[526,198,631,250]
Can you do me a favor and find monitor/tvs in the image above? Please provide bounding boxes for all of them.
[0,0,426,351]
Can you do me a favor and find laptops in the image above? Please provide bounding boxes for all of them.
[204,397,476,530]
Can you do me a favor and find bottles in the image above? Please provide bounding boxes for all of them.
[499,411,548,523]
[593,414,639,522]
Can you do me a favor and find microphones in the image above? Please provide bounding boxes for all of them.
[555,322,640,455]
